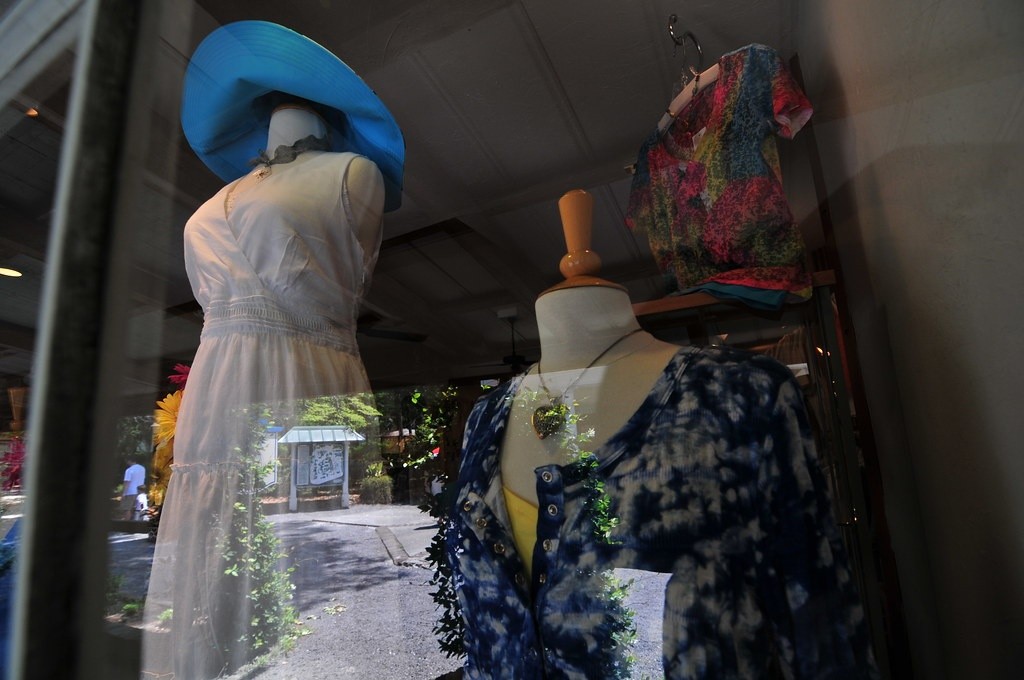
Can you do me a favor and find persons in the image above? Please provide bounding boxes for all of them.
[133,484,148,521]
[137,94,413,680]
[122,456,146,521]
[451,281,864,680]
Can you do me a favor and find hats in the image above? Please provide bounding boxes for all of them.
[180,19,406,213]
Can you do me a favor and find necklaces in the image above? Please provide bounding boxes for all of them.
[532,327,644,441]
[250,136,329,181]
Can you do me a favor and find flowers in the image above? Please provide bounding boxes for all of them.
[148,364,191,508]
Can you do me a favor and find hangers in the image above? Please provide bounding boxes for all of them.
[658,32,720,137]
